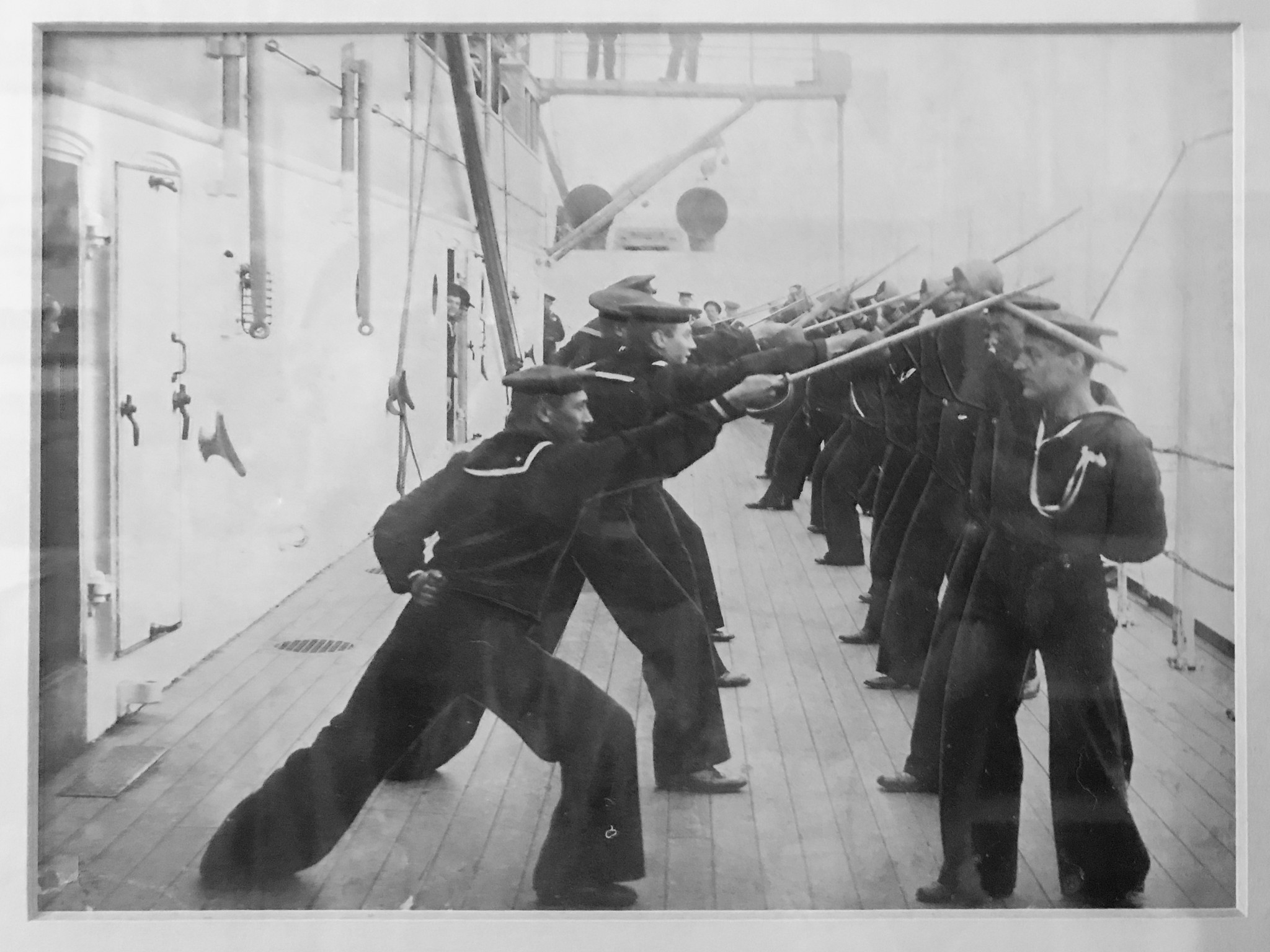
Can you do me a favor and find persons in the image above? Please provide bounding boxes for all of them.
[543,294,565,369]
[876,290,1136,794]
[913,309,1168,908]
[703,300,723,324]
[745,286,1039,699]
[527,276,808,690]
[382,298,891,794]
[677,290,694,307]
[199,365,788,909]
[723,300,741,317]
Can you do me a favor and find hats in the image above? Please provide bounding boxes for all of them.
[608,274,657,294]
[704,301,722,314]
[723,300,740,309]
[501,364,596,394]
[881,299,924,315]
[618,303,702,323]
[544,293,556,301]
[988,291,1061,324]
[1021,309,1118,348]
[678,291,692,298]
[589,287,660,322]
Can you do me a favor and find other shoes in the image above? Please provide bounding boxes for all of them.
[711,630,735,641]
[807,525,826,535]
[814,554,865,566]
[1090,892,1145,909]
[717,670,751,687]
[1022,677,1041,699]
[755,473,770,480]
[838,626,881,645]
[877,771,939,795]
[655,766,747,794]
[858,593,873,602]
[863,674,920,690]
[915,878,1014,904]
[535,883,638,910]
[745,501,793,511]
